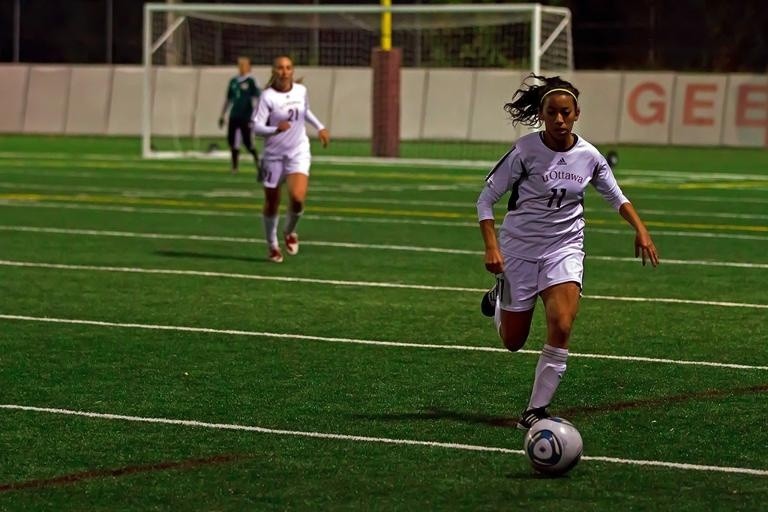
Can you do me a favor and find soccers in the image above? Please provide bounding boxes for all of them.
[524,417,583,474]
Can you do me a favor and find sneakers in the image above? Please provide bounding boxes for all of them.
[481,283,498,317]
[517,404,551,430]
[284,231,299,256]
[268,247,284,263]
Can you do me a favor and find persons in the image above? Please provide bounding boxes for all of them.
[249,54,330,266]
[475,73,660,430]
[217,56,262,175]
